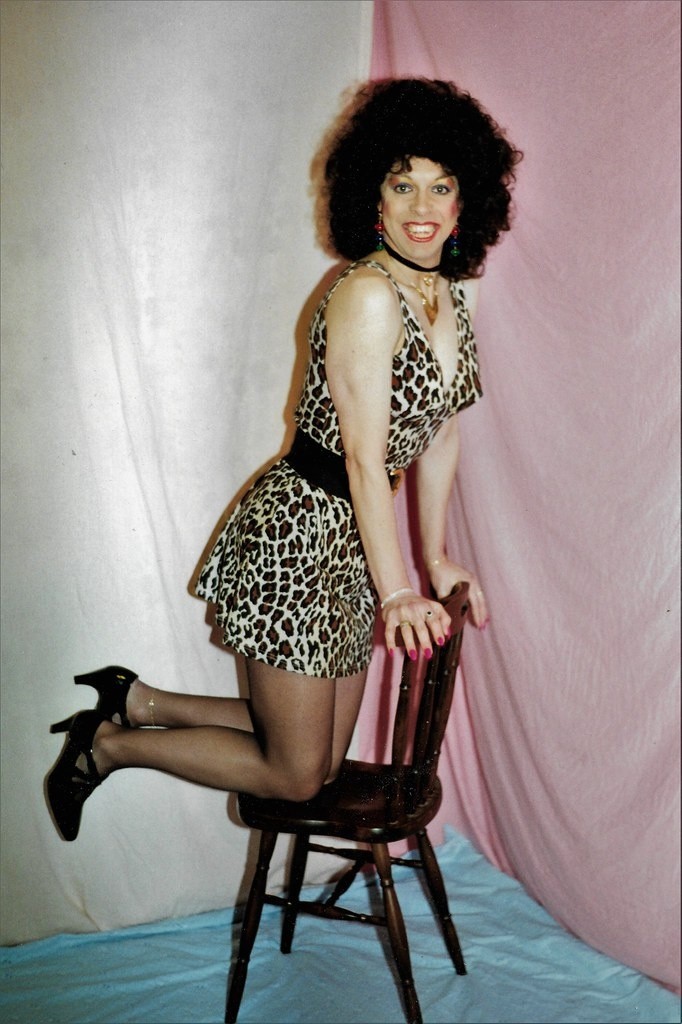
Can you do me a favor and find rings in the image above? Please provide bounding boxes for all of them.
[426,611,433,618]
[400,620,411,628]
[477,591,484,596]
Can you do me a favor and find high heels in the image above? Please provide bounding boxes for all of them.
[43,710,109,842]
[74,666,139,730]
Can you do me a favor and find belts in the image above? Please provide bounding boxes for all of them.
[282,427,405,504]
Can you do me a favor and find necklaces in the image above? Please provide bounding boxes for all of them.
[382,242,441,326]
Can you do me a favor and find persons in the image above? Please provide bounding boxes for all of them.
[43,78,518,842]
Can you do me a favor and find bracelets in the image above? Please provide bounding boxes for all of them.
[380,587,414,609]
[428,555,448,571]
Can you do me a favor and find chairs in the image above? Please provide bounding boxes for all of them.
[223,581,471,1024]
